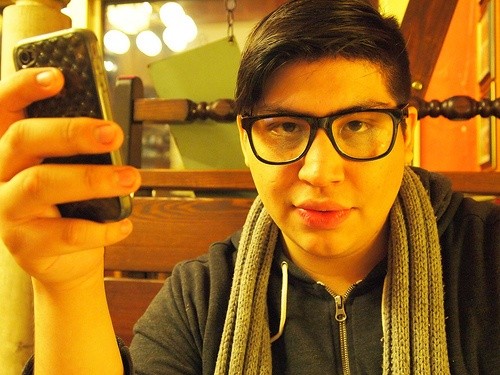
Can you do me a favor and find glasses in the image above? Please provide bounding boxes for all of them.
[242,102,412,165]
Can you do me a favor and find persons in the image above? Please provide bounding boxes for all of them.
[0,0,500,375]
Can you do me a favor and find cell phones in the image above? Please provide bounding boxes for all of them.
[12,28,132,222]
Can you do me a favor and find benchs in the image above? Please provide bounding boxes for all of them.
[103,168,500,349]
